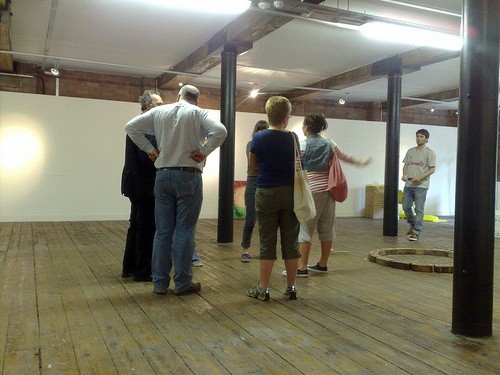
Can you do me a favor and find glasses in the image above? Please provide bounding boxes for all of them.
[288,114,292,119]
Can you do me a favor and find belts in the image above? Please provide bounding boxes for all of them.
[161,167,201,174]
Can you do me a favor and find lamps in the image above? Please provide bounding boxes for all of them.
[338,95,348,104]
[431,106,436,112]
[51,60,61,75]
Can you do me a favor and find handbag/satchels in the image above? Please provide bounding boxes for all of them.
[328,145,349,202]
[290,131,316,223]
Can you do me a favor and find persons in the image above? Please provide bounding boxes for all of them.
[402,130,436,240]
[248,97,301,301]
[282,112,371,278]
[121,91,167,282]
[125,85,227,294]
[240,120,269,262]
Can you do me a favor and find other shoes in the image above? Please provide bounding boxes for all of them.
[246,283,270,301]
[175,282,200,295]
[282,268,308,277]
[284,284,297,298]
[192,260,203,267]
[409,232,418,241]
[240,253,251,262]
[407,229,413,235]
[307,263,328,273]
[153,287,167,295]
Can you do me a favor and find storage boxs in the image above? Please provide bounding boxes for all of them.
[365,184,384,220]
